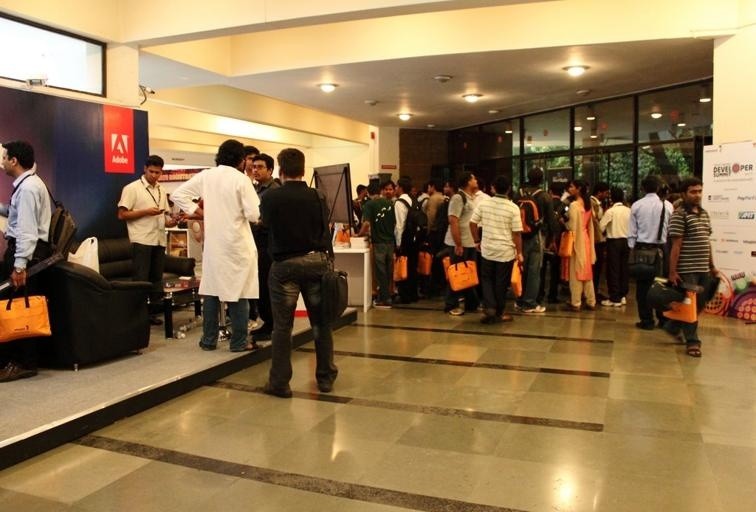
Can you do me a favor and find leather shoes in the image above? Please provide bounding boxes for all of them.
[244,340,259,351]
[150,315,162,325]
[0,362,36,382]
[252,325,273,340]
[636,321,654,330]
[199,338,217,351]
[250,322,269,334]
[480,314,495,323]
[495,313,513,322]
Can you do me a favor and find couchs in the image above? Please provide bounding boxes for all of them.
[69,238,196,319]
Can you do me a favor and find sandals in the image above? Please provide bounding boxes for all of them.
[686,344,701,357]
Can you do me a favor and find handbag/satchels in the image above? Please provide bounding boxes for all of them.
[662,290,697,323]
[557,229,574,258]
[392,255,408,282]
[320,259,349,319]
[417,246,434,275]
[0,284,52,344]
[510,259,524,299]
[442,255,452,281]
[47,205,77,255]
[335,230,348,243]
[646,278,685,311]
[628,248,665,279]
[448,254,480,292]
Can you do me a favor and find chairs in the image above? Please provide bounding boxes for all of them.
[41,262,155,373]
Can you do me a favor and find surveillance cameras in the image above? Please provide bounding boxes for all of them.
[140,84,156,95]
[26,74,49,89]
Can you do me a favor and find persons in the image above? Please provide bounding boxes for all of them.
[444,171,480,315]
[516,168,560,312]
[353,178,459,308]
[630,177,680,329]
[241,144,261,180]
[663,178,721,359]
[470,176,524,323]
[170,137,262,355]
[478,181,529,288]
[115,155,181,328]
[255,147,335,398]
[248,151,283,343]
[1,139,56,385]
[535,182,632,312]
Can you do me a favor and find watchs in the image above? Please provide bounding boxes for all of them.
[14,267,27,274]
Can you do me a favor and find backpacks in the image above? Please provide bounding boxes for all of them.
[515,187,547,240]
[393,194,428,247]
[435,191,466,234]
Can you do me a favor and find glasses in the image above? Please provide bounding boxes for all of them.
[250,164,268,170]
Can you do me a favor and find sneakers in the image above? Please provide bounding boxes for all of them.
[523,303,546,313]
[601,298,622,307]
[264,378,292,398]
[514,302,522,312]
[372,302,391,309]
[448,306,465,316]
[621,296,626,305]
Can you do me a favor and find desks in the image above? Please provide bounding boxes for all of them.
[324,245,373,313]
[164,227,202,264]
